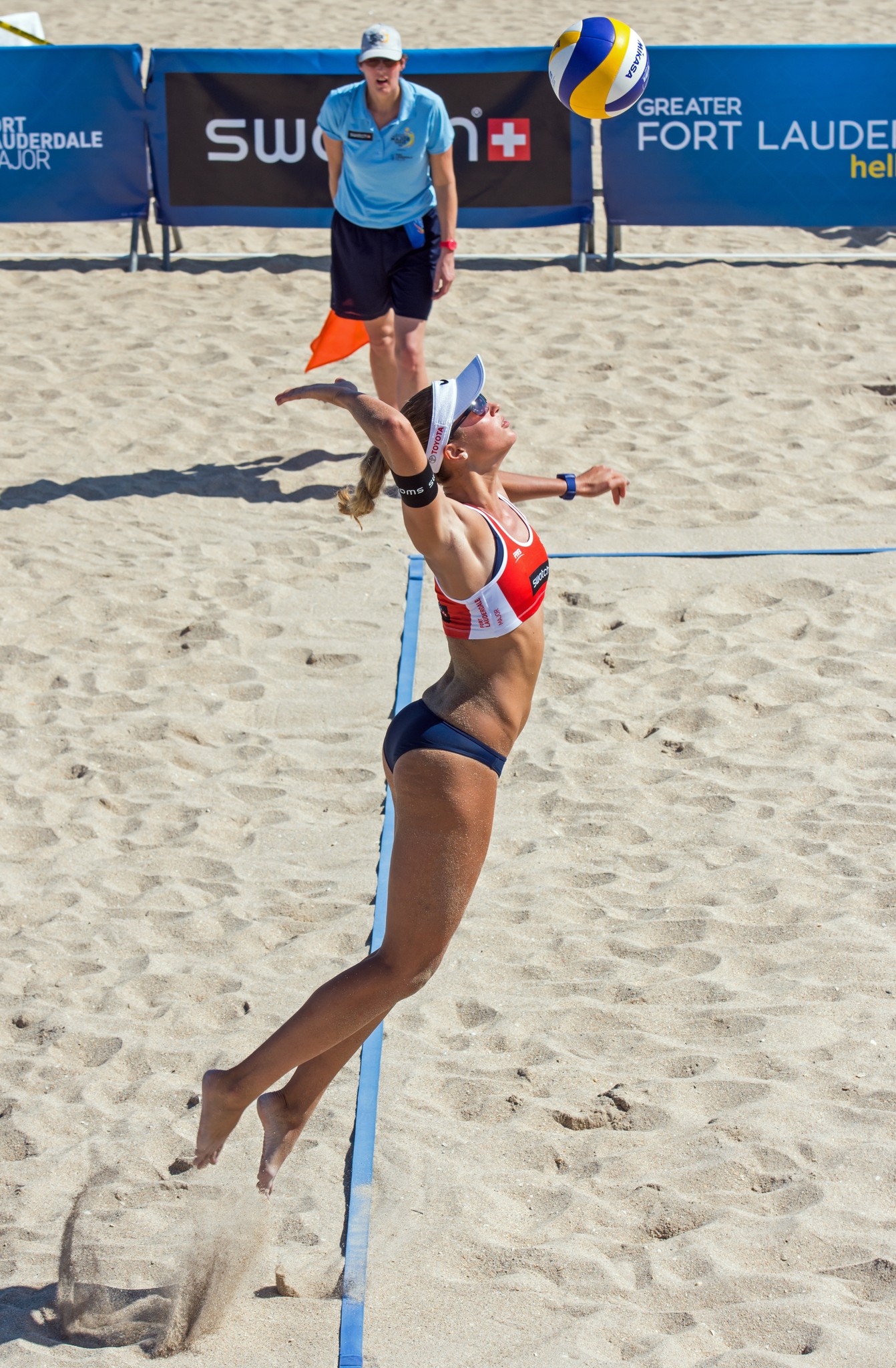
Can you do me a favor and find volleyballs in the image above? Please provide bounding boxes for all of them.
[548,16,651,120]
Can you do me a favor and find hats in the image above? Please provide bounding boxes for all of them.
[358,23,403,62]
[426,353,485,474]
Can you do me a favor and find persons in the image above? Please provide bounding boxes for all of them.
[188,356,631,1194]
[315,24,460,414]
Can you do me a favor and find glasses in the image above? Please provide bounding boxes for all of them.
[448,393,488,444]
[364,58,400,69]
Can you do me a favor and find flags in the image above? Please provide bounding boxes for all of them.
[305,308,371,373]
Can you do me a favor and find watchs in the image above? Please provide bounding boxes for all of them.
[558,473,576,501]
[440,239,457,251]
